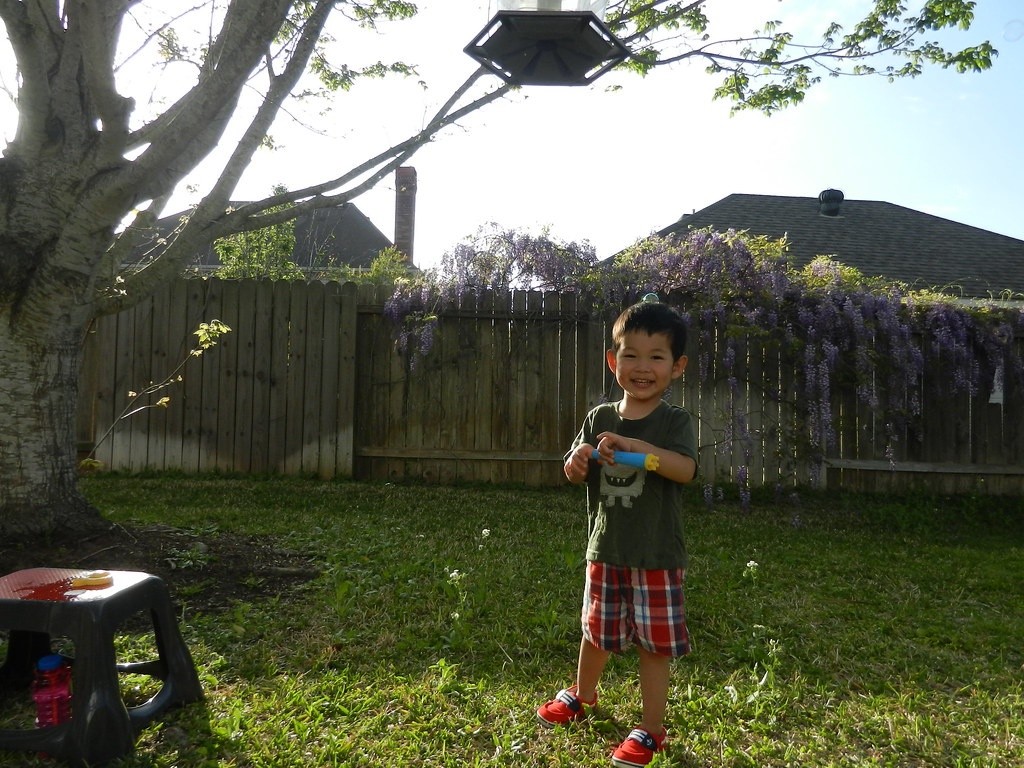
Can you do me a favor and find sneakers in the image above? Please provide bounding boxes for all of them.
[612,724,668,768]
[536,685,597,729]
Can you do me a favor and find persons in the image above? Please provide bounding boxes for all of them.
[533,299,704,761]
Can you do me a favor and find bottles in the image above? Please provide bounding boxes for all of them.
[33,655,71,730]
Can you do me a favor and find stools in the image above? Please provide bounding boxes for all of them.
[1,567,207,768]
